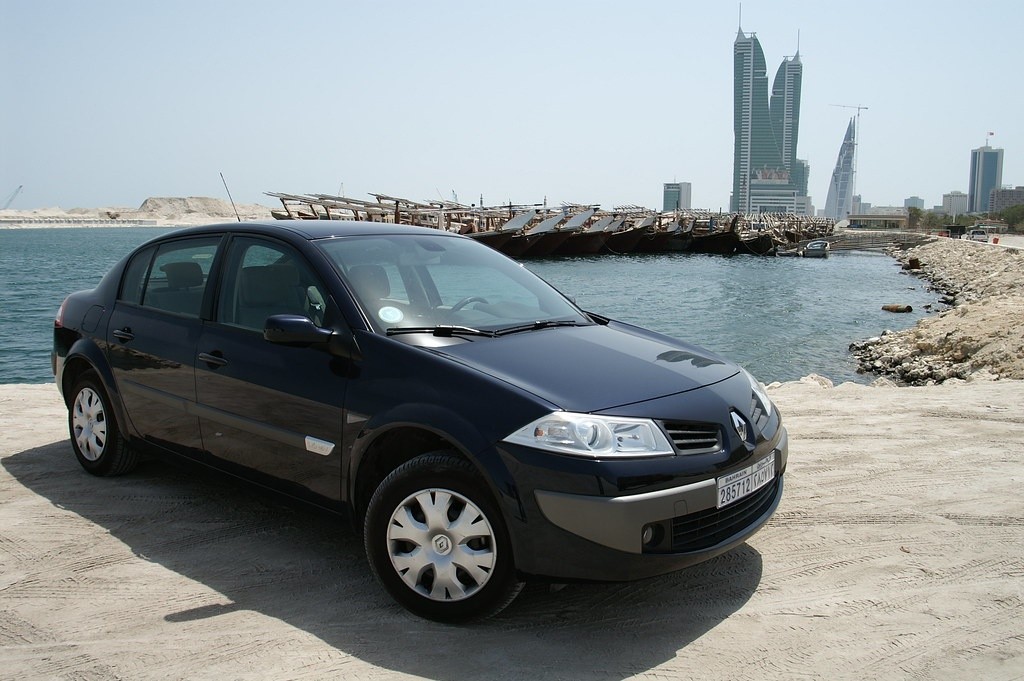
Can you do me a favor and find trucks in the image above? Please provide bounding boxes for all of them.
[966,230,989,242]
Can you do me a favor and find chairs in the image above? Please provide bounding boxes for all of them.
[236,266,306,329]
[347,265,413,331]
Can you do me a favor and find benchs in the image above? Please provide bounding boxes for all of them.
[151,261,309,320]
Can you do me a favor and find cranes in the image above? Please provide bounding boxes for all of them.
[828,103,869,214]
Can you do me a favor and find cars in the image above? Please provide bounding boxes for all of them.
[51,221,789,626]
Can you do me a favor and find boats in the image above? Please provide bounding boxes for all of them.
[802,241,830,259]
[262,191,838,257]
